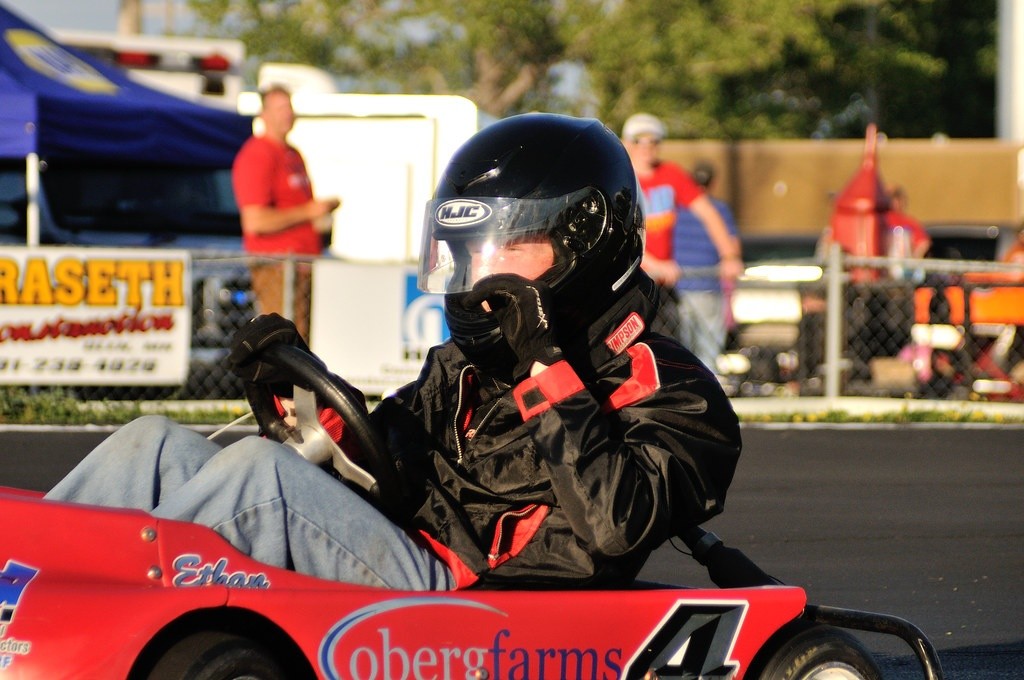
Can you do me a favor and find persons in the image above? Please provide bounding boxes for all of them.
[673,163,742,374]
[44,113,742,590]
[233,86,340,344]
[885,186,932,257]
[622,112,743,340]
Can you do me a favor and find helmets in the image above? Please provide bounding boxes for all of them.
[432,113,647,379]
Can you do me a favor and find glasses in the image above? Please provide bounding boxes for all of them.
[631,138,659,145]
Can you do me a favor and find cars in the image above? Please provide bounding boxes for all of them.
[912,221,1023,373]
[728,232,832,386]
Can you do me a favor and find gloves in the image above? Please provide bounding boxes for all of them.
[465,274,566,380]
[224,312,327,393]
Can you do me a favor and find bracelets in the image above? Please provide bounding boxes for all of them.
[721,254,740,261]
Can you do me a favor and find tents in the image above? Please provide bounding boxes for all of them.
[0,4,253,246]
